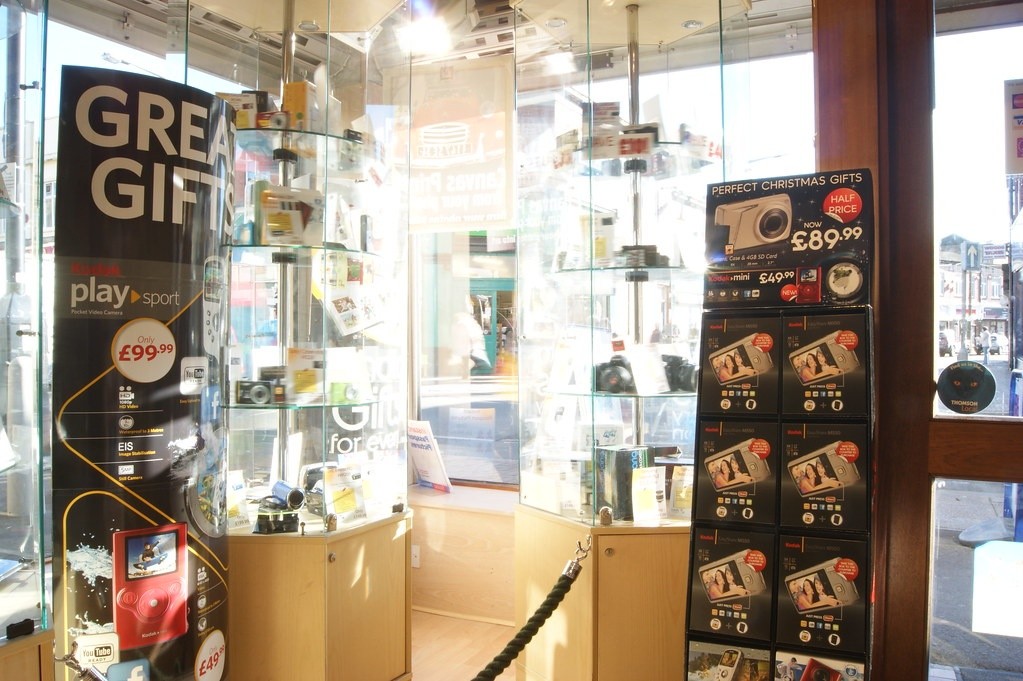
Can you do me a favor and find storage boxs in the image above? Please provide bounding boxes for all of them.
[774,640,872,681]
[776,533,875,655]
[691,416,779,528]
[684,632,774,681]
[697,311,783,416]
[685,524,778,643]
[782,310,873,420]
[704,169,873,309]
[782,421,873,534]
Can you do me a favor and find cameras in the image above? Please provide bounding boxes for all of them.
[272,480,305,511]
[236,380,273,405]
[699,194,861,681]
[661,354,700,393]
[256,111,289,129]
[594,354,638,393]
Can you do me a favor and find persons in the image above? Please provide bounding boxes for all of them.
[451,293,492,378]
[791,460,840,493]
[712,352,751,381]
[792,350,840,383]
[981,327,991,366]
[788,575,838,611]
[708,455,752,489]
[702,565,747,600]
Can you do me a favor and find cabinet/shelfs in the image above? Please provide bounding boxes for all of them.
[516,140,723,681]
[224,126,412,681]
